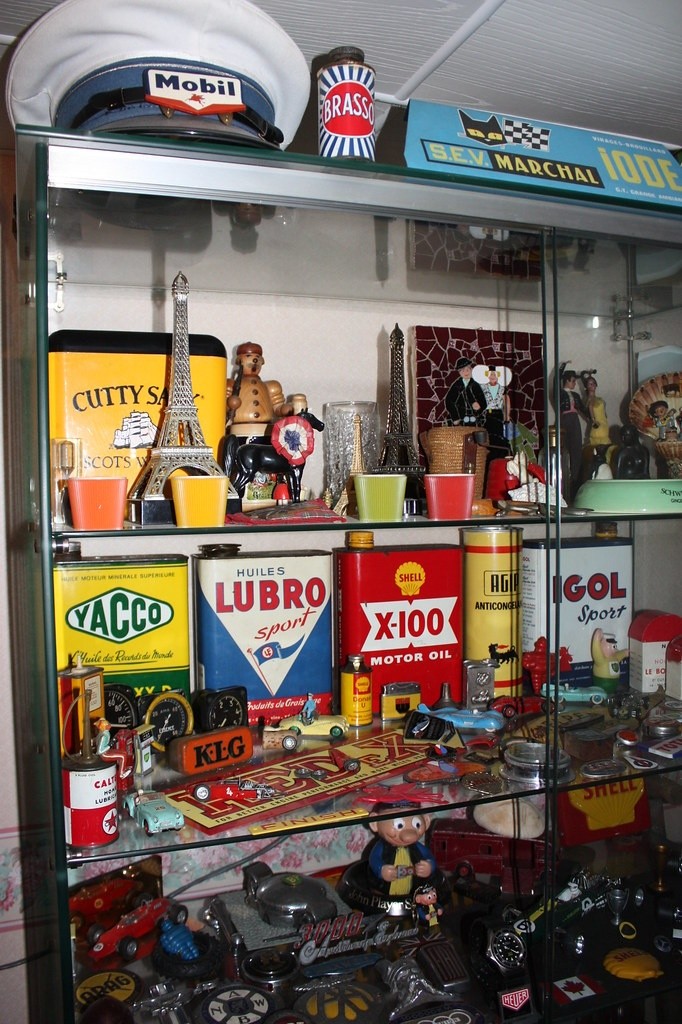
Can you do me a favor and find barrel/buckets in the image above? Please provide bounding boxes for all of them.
[522,520,634,700]
[192,542,335,726]
[53,541,190,706]
[332,532,464,718]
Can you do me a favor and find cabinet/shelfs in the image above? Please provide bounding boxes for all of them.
[15,123,682,1023]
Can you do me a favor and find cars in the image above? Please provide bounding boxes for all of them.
[88,898,189,964]
[490,695,555,719]
[260,708,349,752]
[188,775,275,802]
[122,789,185,837]
[540,683,608,704]
[418,703,506,733]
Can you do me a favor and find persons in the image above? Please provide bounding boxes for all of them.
[553,361,598,477]
[414,883,443,931]
[612,425,652,480]
[649,401,677,442]
[581,369,611,446]
[359,801,440,894]
[302,693,316,718]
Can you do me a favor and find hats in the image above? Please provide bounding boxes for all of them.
[5,0,311,152]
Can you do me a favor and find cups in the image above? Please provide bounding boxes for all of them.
[170,475,230,528]
[68,477,128,529]
[459,525,521,697]
[353,473,407,523]
[423,472,476,519]
[324,402,382,510]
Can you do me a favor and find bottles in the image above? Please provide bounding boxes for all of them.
[340,655,373,726]
[538,427,569,499]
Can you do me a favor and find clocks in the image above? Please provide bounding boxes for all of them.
[103,684,249,756]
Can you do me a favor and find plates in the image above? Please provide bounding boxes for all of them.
[573,480,681,516]
